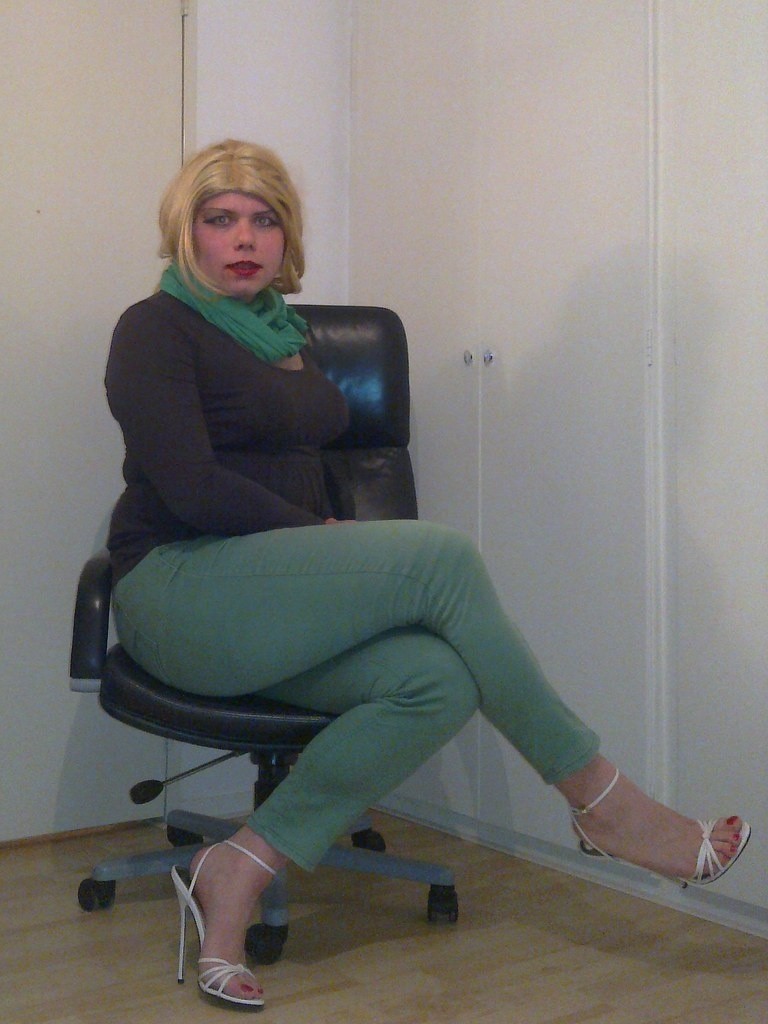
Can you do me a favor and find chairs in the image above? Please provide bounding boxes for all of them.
[68,304,459,966]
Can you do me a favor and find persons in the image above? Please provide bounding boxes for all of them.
[105,142,753,1003]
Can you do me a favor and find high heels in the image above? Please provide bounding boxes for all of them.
[171,839,276,1007]
[569,768,751,888]
